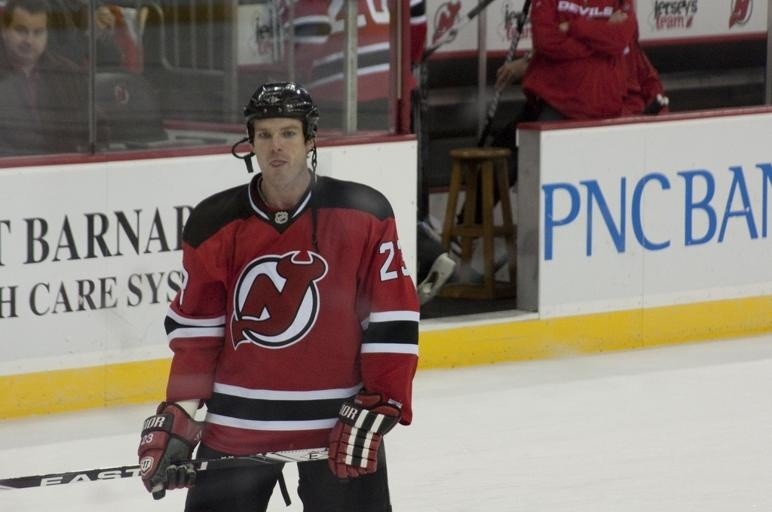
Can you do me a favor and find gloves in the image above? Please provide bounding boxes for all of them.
[328,393,401,478]
[138,402,205,499]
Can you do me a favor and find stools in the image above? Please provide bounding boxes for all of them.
[432,146,516,301]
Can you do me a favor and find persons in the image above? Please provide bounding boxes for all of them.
[134,79,421,510]
[0,0,230,158]
[453,2,673,285]
[285,2,457,316]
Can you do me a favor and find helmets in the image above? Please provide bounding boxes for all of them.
[244,81,319,143]
